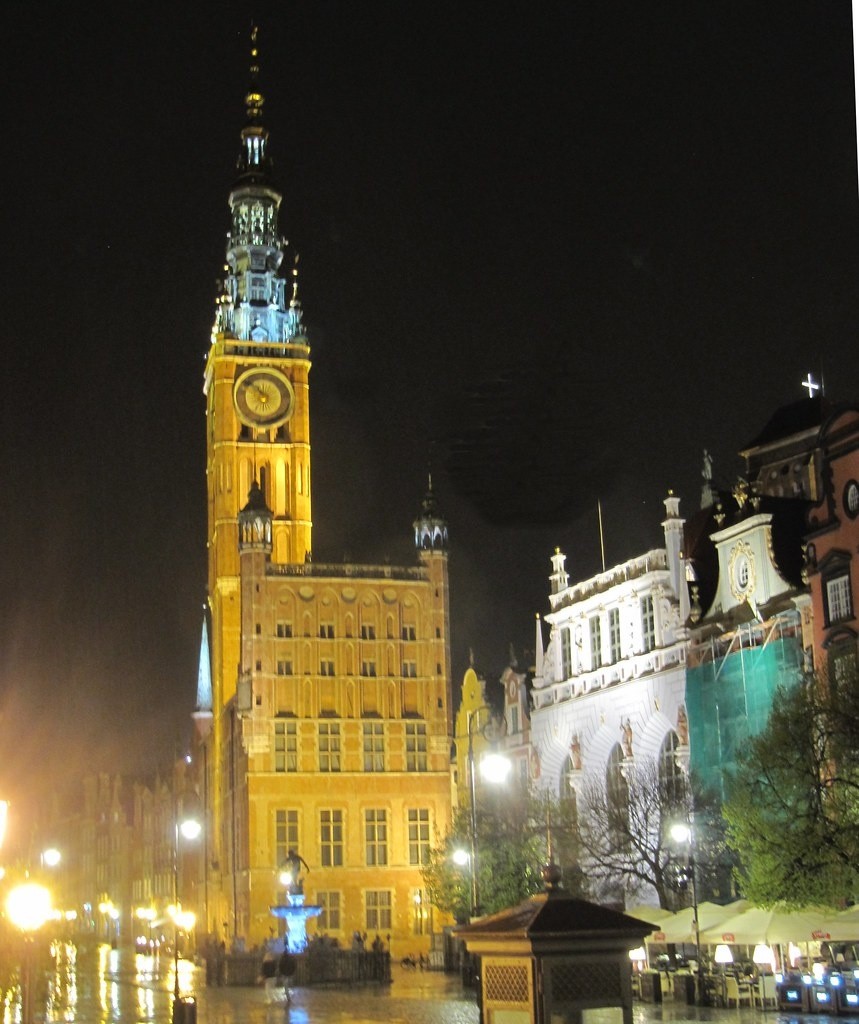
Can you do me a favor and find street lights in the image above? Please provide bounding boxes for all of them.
[451,703,508,987]
[174,788,202,1004]
[666,821,708,1006]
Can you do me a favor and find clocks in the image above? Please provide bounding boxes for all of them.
[235,373,292,424]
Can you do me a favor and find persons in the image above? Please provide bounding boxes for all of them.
[279,850,310,887]
[198,940,227,988]
[260,950,295,1005]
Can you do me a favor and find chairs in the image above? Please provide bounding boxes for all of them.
[706,971,779,1010]
